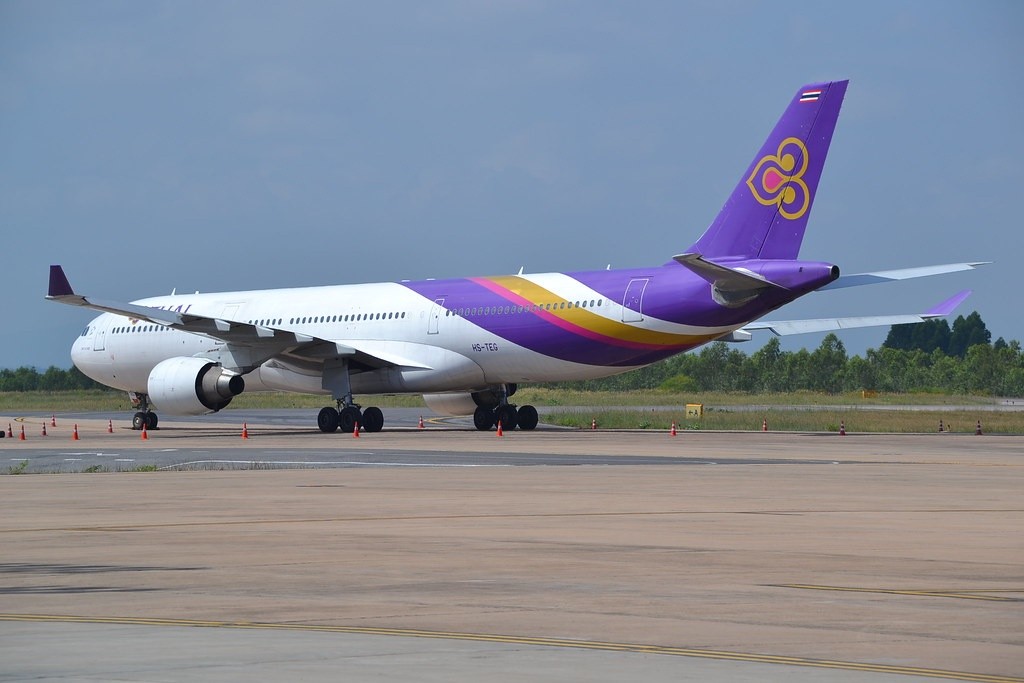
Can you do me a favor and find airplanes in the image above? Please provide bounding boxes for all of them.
[37,77,996,435]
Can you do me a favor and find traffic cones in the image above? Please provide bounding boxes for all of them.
[590,416,597,429]
[41,422,47,436]
[974,420,983,435]
[6,423,13,438]
[762,417,767,432]
[839,420,846,436]
[670,419,677,436]
[418,415,425,428]
[352,420,360,438]
[938,419,944,433]
[72,424,80,440]
[495,420,503,436]
[141,423,148,440]
[50,414,56,427]
[242,422,248,440]
[18,424,26,440]
[107,419,113,433]
